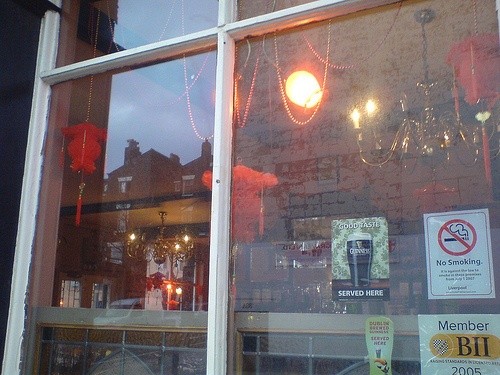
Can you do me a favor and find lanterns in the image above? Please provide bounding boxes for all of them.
[444,33,500,181]
[144,276,154,305]
[202,163,279,309]
[150,264,166,307]
[61,122,108,227]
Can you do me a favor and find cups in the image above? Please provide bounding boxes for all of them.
[346,232,374,288]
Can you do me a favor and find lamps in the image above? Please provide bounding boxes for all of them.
[126,211,196,266]
[260,35,322,109]
[348,8,500,176]
